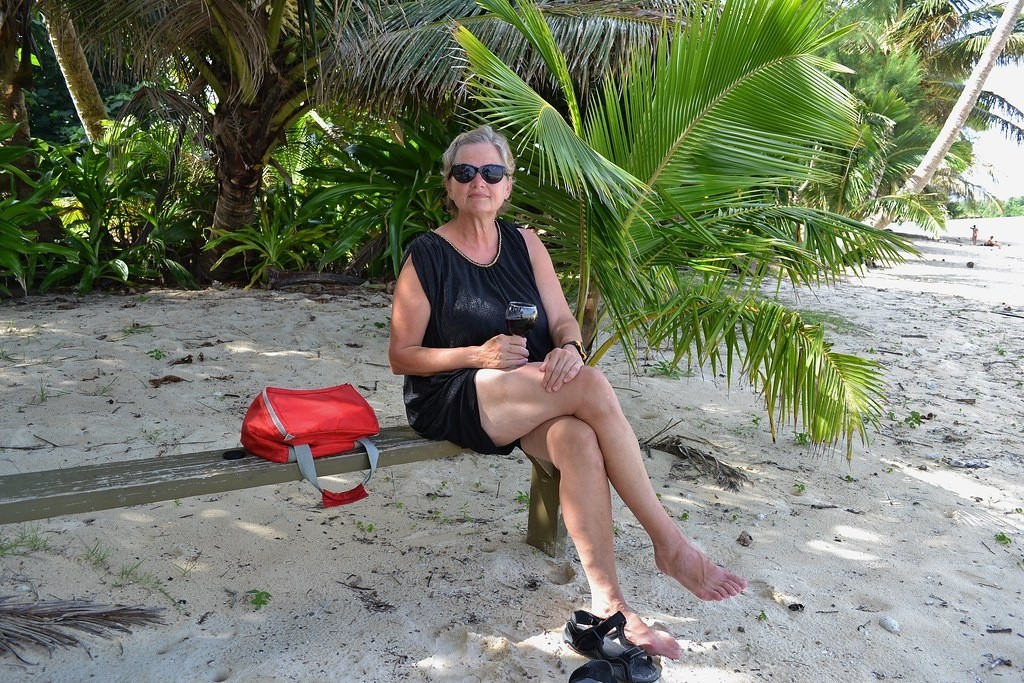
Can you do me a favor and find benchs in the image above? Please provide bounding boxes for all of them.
[0,423,568,558]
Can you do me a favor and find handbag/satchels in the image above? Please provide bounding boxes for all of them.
[240,382,381,510]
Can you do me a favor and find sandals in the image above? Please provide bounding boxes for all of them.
[567,659,616,683]
[562,608,664,683]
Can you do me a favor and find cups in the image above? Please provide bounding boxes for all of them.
[505,302,538,337]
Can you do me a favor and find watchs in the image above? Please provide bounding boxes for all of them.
[562,340,589,364]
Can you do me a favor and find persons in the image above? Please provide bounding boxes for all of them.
[984,235,1001,249]
[387,123,749,659]
[970,225,979,245]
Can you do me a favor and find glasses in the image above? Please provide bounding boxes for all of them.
[447,163,510,185]
